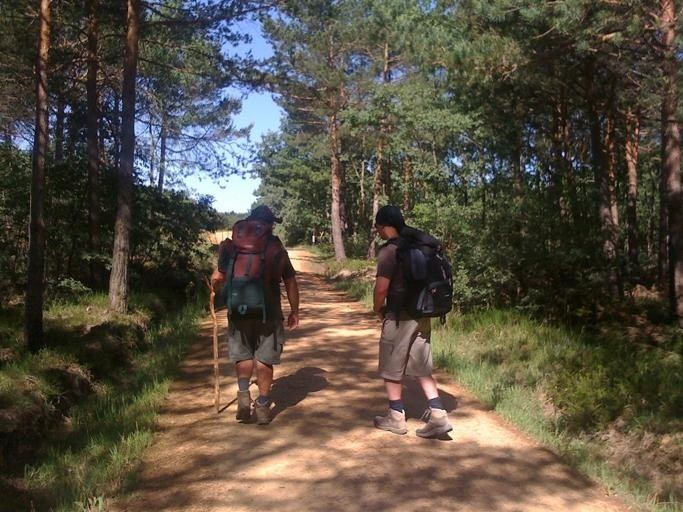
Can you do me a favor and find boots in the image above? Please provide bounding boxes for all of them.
[416,406,452,438]
[236,389,251,420]
[255,399,273,425]
[374,407,408,435]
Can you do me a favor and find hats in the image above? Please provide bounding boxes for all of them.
[249,204,283,224]
[376,205,405,227]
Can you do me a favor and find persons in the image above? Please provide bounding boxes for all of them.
[210,206,299,424]
[372,205,454,439]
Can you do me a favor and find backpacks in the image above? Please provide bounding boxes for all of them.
[386,232,453,318]
[223,218,285,334]
